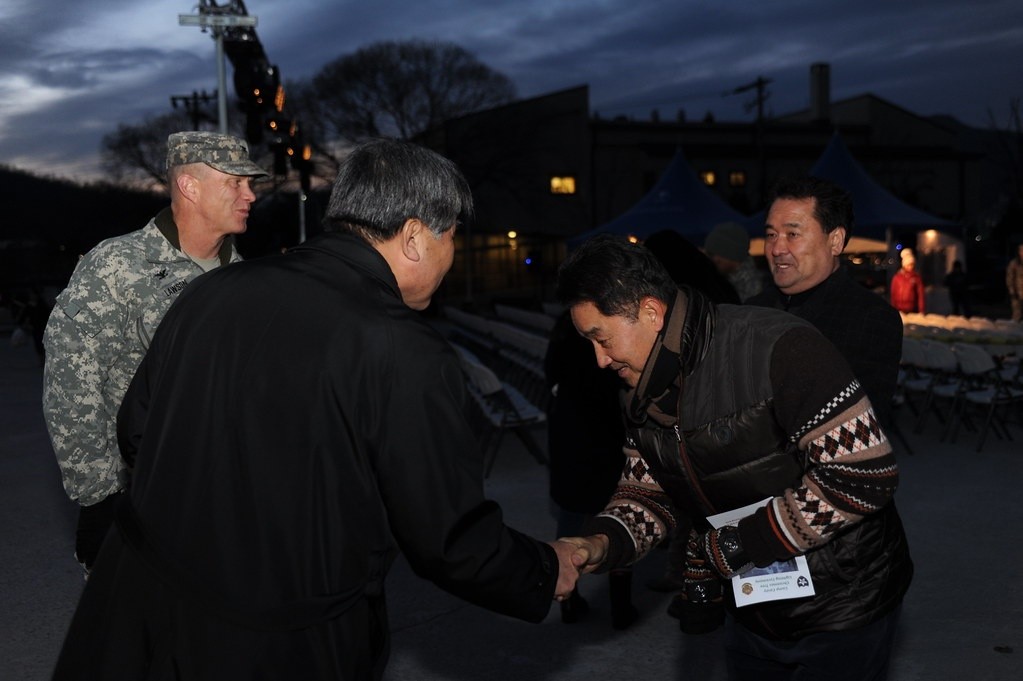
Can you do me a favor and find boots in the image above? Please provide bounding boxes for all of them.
[609,570,638,631]
[561,581,589,624]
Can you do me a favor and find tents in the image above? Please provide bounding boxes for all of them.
[568,150,765,254]
[752,129,945,233]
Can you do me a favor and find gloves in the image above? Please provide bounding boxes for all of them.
[667,529,727,635]
[696,513,777,583]
[76,490,122,569]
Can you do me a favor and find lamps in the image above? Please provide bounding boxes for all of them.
[222,29,314,182]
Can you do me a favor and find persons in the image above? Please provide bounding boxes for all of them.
[538,173,1023,681]
[41,130,269,580]
[52,137,579,681]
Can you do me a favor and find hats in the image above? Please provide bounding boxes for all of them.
[166,131,269,178]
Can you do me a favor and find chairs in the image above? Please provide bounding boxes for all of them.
[435,295,571,475]
[889,309,1023,458]
